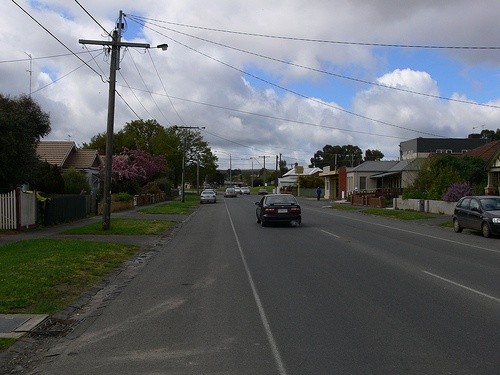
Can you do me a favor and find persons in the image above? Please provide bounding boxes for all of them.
[316,186,322,200]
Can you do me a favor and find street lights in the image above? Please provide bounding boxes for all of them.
[250,157,265,188]
[102,43,169,232]
[215,150,231,188]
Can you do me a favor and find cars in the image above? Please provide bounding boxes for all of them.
[254,193,302,227]
[200,189,217,204]
[225,186,250,198]
[257,188,268,196]
[452,195,500,240]
